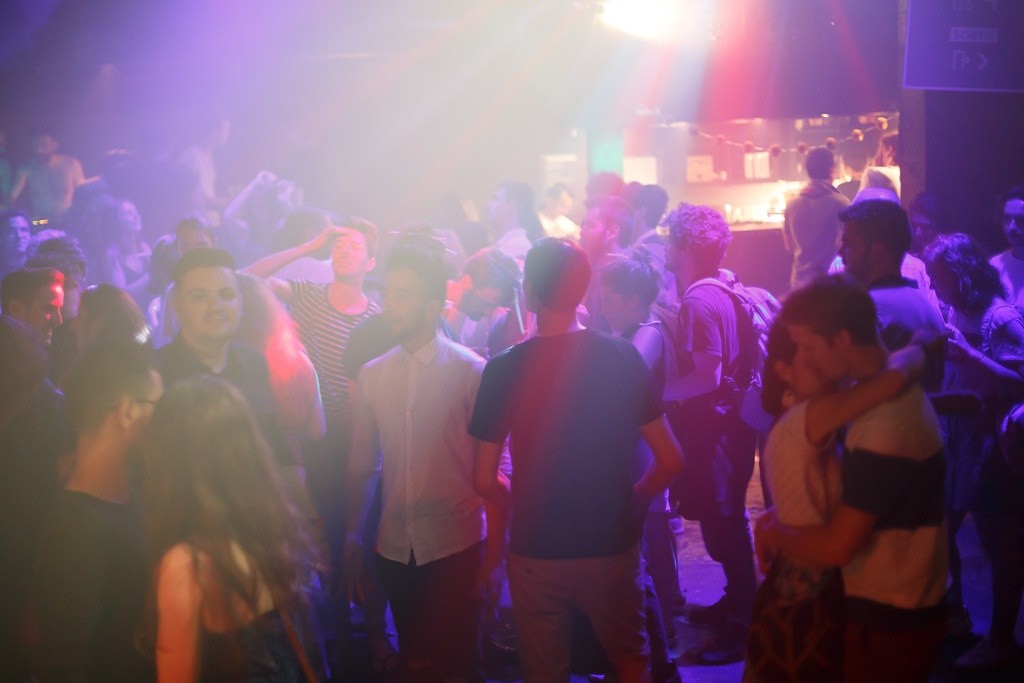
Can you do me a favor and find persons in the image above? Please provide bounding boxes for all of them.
[1,127,1024,683]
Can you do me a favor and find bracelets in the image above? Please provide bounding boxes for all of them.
[975,351,984,361]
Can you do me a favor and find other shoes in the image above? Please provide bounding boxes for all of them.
[410,657,436,672]
[696,624,751,663]
[691,595,730,624]
[953,638,1020,669]
[942,603,974,636]
[369,644,399,675]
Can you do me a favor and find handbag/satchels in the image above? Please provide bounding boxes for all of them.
[981,307,1024,475]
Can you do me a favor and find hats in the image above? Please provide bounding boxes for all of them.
[346,216,383,276]
[36,237,86,267]
[837,187,901,222]
[26,229,65,261]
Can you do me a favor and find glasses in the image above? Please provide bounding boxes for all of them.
[180,288,242,301]
[334,239,368,252]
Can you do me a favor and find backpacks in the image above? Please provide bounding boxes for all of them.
[682,277,777,431]
